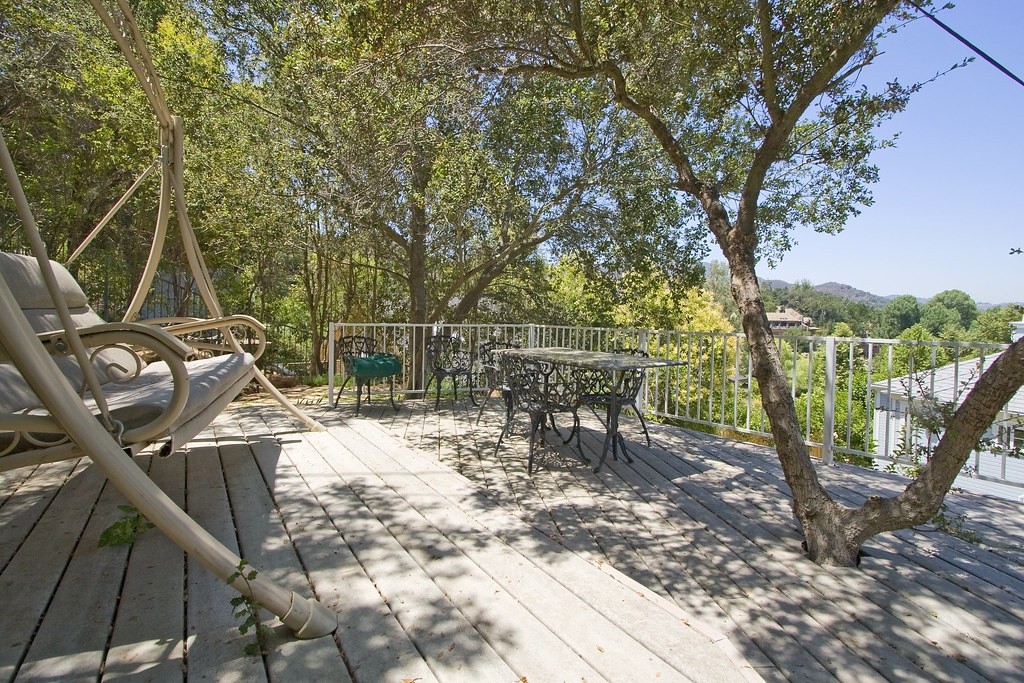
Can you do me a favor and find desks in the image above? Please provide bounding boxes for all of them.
[492,346,688,474]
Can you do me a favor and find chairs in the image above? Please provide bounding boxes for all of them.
[476,341,562,438]
[494,354,591,477]
[334,336,399,417]
[423,336,480,410]
[563,348,651,447]
